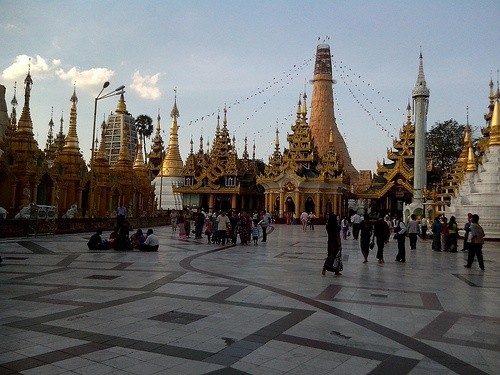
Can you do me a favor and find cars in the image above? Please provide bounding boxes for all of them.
[0,207,9,220]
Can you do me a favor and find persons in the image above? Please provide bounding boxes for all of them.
[169,205,279,246]
[322,210,486,275]
[86,201,160,251]
[286,210,317,231]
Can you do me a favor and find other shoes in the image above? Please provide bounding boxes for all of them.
[322,269,325,275]
[399,260,406,263]
[464,264,471,268]
[480,268,485,271]
[335,272,342,275]
[381,260,384,263]
[378,259,382,262]
[366,260,368,262]
[461,249,465,251]
[362,261,366,263]
[395,259,400,262]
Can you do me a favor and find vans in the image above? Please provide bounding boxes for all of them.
[15,203,58,218]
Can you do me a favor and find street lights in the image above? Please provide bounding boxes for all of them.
[421,192,428,217]
[88,79,126,232]
